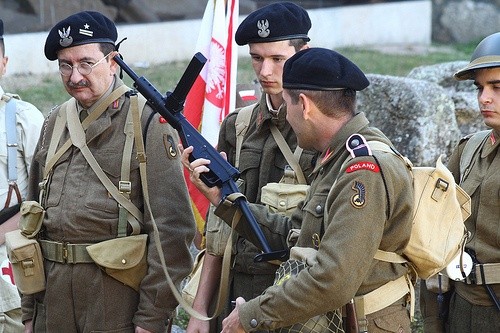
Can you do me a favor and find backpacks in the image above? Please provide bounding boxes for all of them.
[324,141,473,279]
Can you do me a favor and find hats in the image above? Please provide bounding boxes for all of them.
[44,10,118,60]
[236,1,311,46]
[283,47,369,92]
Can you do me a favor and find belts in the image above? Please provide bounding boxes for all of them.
[461,263,500,284]
[340,275,410,318]
[37,240,96,264]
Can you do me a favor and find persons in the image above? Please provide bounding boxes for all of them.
[17,11,196,333]
[0,18,47,333]
[185,2,311,333]
[420,30,500,333]
[176,47,414,333]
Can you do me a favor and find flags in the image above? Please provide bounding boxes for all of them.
[182,0,239,252]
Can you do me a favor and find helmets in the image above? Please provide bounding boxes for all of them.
[453,33,500,82]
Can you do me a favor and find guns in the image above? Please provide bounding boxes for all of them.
[113,51,287,265]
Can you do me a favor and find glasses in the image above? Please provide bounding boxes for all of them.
[58,51,112,76]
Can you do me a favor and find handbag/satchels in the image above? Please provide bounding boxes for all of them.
[206,203,238,256]
[86,235,147,291]
[5,229,47,295]
[260,182,310,218]
